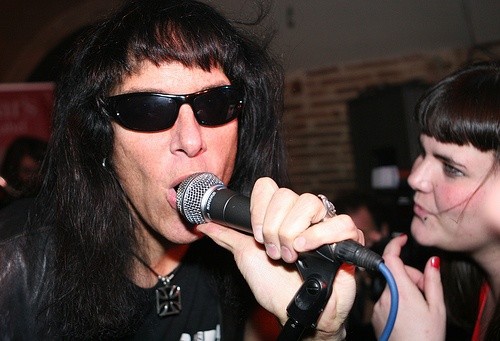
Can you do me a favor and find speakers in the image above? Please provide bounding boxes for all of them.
[348,80,435,195]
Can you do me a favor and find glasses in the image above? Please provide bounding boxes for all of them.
[104,85,242,133]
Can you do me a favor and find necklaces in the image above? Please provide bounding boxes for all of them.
[121,246,182,316]
[317,194,337,220]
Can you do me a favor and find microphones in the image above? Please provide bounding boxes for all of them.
[175,173,385,272]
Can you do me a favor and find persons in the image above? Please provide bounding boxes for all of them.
[371,62,500,341]
[0,0,366,341]
[0,136,394,341]
